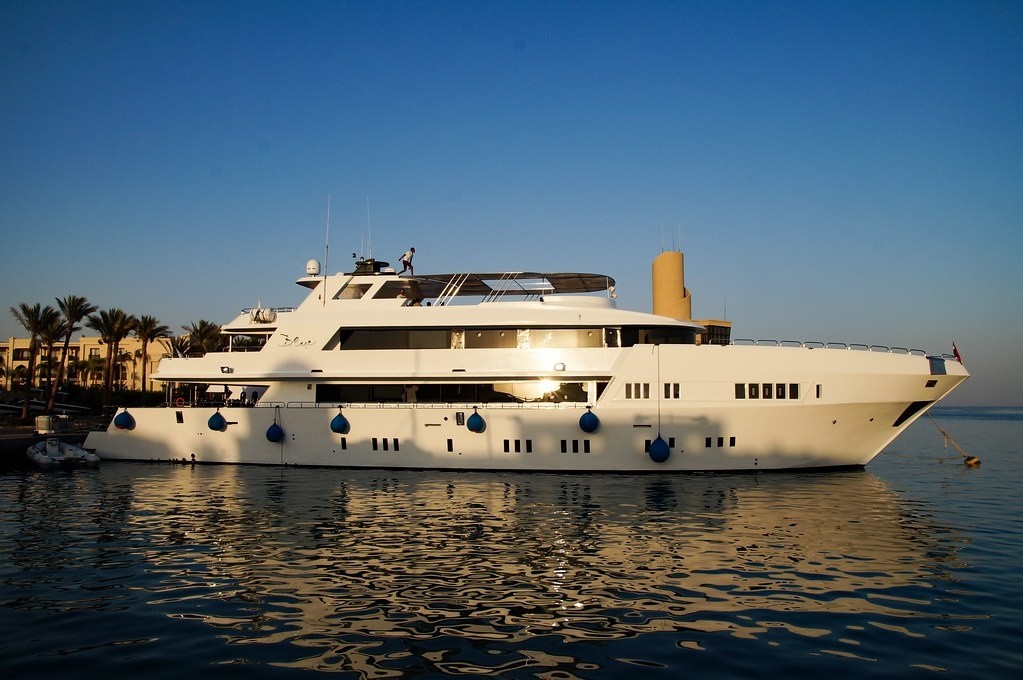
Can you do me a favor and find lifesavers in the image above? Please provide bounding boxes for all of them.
[175,397,185,407]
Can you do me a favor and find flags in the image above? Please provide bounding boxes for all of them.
[953,341,963,365]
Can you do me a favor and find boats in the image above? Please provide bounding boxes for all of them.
[27,438,100,469]
[83,194,971,473]
[0,389,90,427]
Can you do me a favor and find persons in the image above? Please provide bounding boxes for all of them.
[63,379,71,393]
[113,380,119,391]
[396,247,415,276]
[397,289,407,299]
[240,390,258,404]
